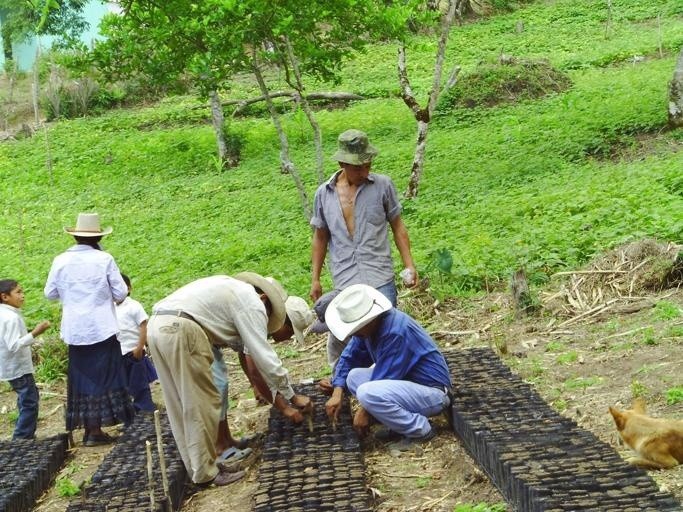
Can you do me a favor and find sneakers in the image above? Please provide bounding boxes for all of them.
[83,433,116,446]
[397,430,435,451]
[376,426,401,441]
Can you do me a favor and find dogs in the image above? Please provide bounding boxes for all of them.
[608,397,683,469]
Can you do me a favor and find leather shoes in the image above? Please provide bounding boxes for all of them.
[202,461,246,485]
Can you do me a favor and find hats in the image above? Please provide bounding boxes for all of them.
[332,129,379,166]
[235,272,287,332]
[63,213,113,237]
[325,283,392,341]
[310,290,341,333]
[285,296,313,345]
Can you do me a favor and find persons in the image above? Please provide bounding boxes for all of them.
[44,213,135,446]
[310,129,417,309]
[306,291,351,395]
[209,295,317,464]
[324,284,454,451]
[107,273,158,416]
[147,272,315,489]
[0,279,52,441]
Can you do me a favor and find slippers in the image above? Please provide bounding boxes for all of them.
[217,438,252,462]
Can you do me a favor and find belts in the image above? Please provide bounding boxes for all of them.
[152,310,194,320]
